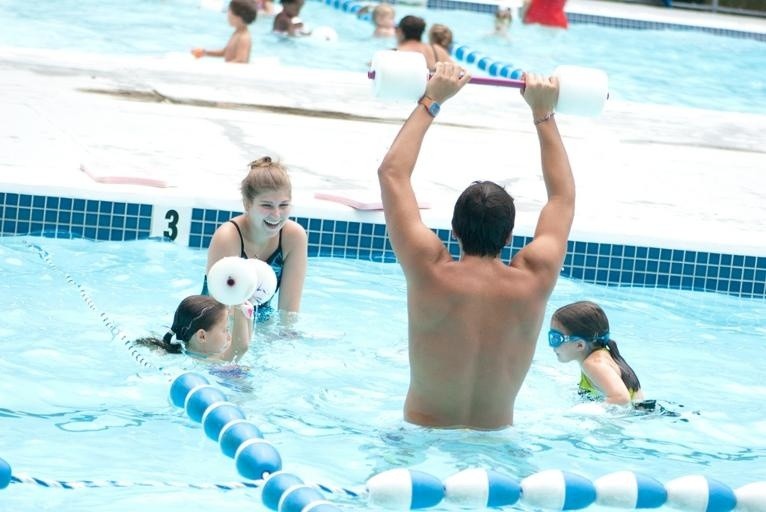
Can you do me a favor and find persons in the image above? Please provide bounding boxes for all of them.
[364,16,438,72]
[376,59,579,431]
[519,0,569,30]
[191,0,256,65]
[272,0,307,39]
[132,294,256,384]
[255,0,276,14]
[548,299,704,424]
[428,23,453,71]
[474,8,515,54]
[200,156,308,343]
[371,3,396,37]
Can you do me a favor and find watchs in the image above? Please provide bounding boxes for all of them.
[417,93,442,119]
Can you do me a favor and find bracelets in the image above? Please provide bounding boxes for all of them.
[531,110,557,129]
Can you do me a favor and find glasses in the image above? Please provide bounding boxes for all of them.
[548,330,583,347]
